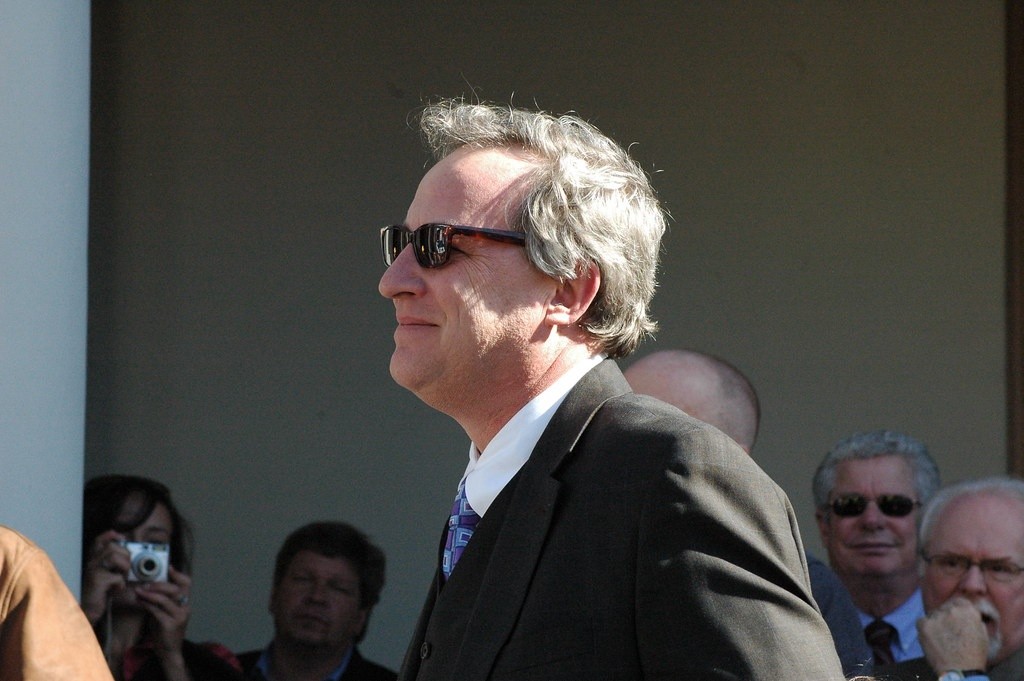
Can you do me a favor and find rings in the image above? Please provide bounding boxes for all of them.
[178,593,189,605]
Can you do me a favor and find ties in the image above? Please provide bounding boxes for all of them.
[443,483,480,582]
[863,621,898,667]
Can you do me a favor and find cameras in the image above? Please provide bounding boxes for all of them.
[115,540,169,583]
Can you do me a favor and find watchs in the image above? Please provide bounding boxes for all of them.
[937,669,984,681]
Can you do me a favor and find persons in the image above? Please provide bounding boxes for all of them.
[82,474,244,681]
[378,102,845,681]
[624,347,1024,681]
[236,521,398,681]
[0,525,115,681]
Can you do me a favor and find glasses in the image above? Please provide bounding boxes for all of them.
[822,492,923,519]
[380,222,527,269]
[920,551,1024,583]
